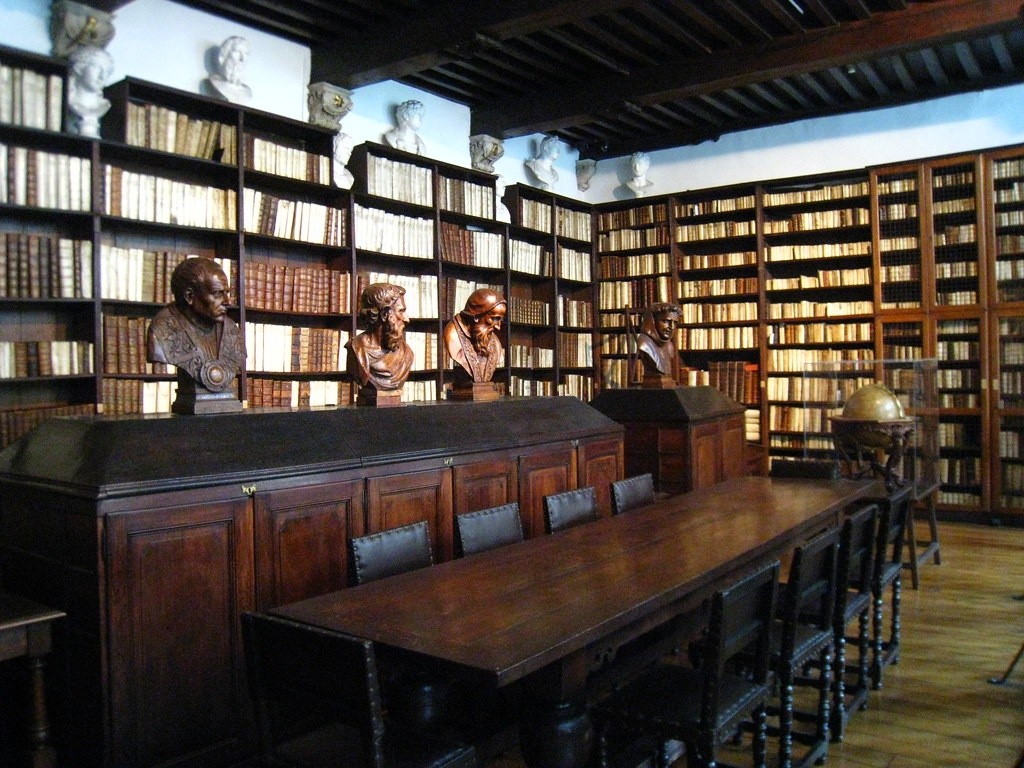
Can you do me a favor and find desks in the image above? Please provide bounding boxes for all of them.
[904,480,945,589]
[256,470,889,768]
[0,588,77,768]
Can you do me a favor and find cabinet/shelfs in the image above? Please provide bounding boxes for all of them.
[0,77,1024,556]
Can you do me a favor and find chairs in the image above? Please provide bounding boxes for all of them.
[241,473,915,767]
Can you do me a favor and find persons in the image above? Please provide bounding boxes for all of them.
[344,283,414,390]
[383,100,425,156]
[443,290,506,382]
[626,152,653,193]
[333,133,354,190]
[637,303,683,375]
[526,136,558,185]
[62,45,114,140]
[208,36,256,109]
[147,258,247,392]
[492,174,512,224]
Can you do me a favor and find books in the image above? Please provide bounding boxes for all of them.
[354,151,1024,510]
[0,65,352,450]
